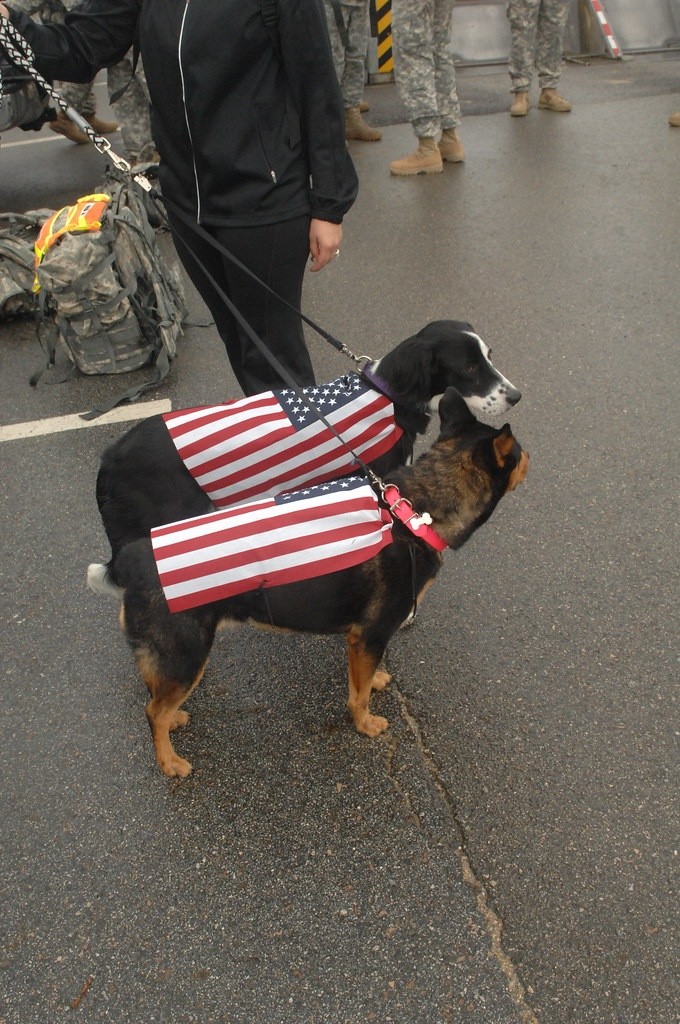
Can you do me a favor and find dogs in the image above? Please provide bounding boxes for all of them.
[88,386,530,777]
[95,320,522,601]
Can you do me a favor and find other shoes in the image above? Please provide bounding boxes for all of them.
[117,151,161,180]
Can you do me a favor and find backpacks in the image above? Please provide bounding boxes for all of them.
[0,45,59,131]
[31,183,191,375]
[0,208,66,320]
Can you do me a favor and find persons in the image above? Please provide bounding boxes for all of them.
[3,0,161,185]
[325,0,573,174]
[0,0,359,397]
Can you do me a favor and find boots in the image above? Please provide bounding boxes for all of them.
[343,103,383,140]
[50,109,89,144]
[538,88,572,112]
[510,91,531,116]
[359,100,370,112]
[668,112,680,127]
[81,114,119,134]
[388,137,443,176]
[438,127,464,163]
